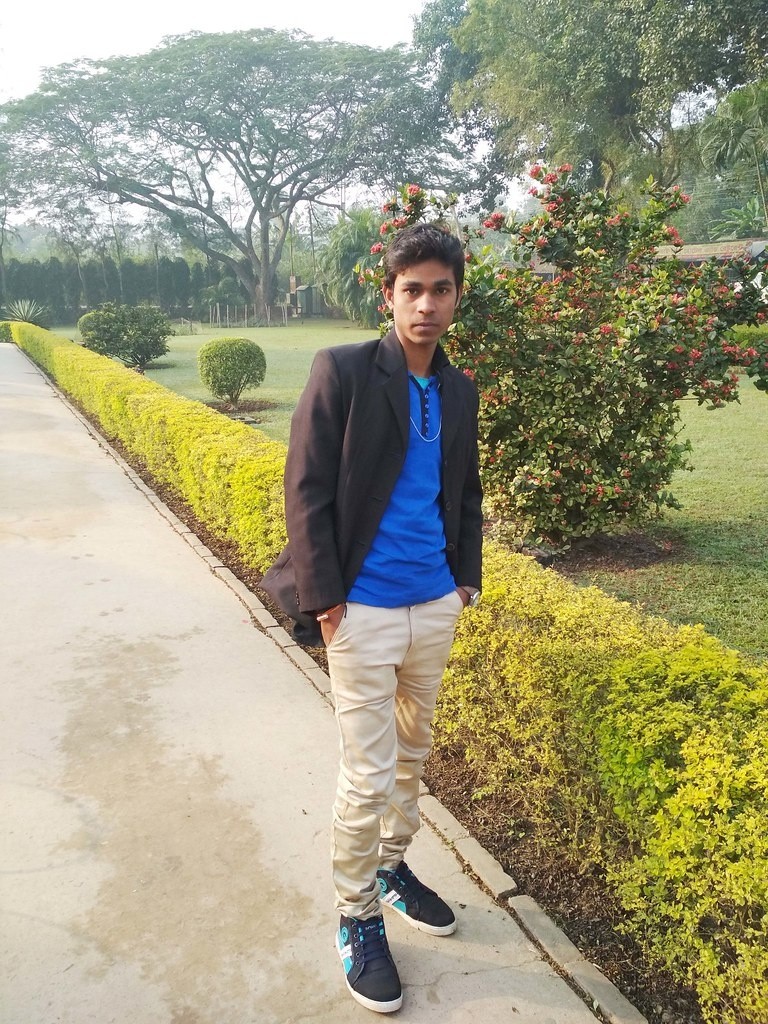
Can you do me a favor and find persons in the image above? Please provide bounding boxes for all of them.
[256,223,483,1015]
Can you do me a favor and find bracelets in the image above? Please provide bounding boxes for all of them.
[314,601,347,622]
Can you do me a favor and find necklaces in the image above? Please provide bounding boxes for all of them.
[405,391,443,442]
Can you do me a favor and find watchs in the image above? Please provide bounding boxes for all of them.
[458,583,481,607]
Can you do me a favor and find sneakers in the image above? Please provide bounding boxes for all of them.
[377,860,456,936]
[335,913,403,1013]
